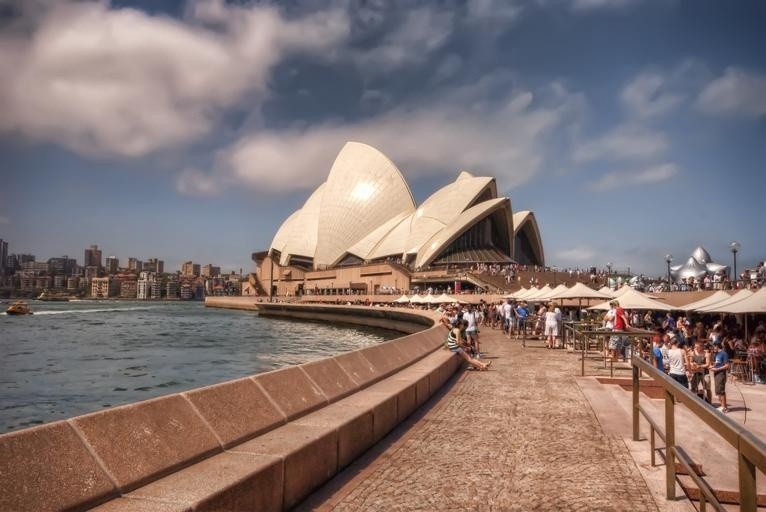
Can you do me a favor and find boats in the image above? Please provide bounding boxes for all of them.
[35,287,70,302]
[6,300,33,316]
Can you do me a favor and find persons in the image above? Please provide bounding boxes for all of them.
[343,263,604,370]
[604,262,765,413]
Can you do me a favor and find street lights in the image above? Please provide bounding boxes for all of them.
[331,273,428,293]
[463,271,468,283]
[605,261,613,288]
[729,240,740,288]
[664,252,672,290]
[552,264,558,287]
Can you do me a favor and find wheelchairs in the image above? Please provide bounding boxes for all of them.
[482,315,498,329]
